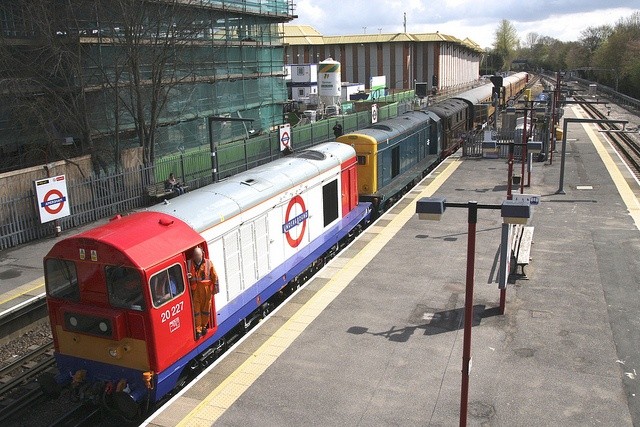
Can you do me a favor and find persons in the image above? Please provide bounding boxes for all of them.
[332,120,343,138]
[167,172,185,196]
[123,274,179,307]
[186,246,220,341]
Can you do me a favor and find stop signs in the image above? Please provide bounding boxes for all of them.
[34,174,70,224]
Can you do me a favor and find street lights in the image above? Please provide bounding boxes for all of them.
[517,100,547,138]
[481,141,542,315]
[415,197,529,427]
[542,90,568,165]
[507,107,545,194]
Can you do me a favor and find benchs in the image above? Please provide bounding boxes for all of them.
[509,220,536,280]
[146,176,190,204]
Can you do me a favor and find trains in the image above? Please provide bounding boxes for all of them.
[38,70,529,423]
